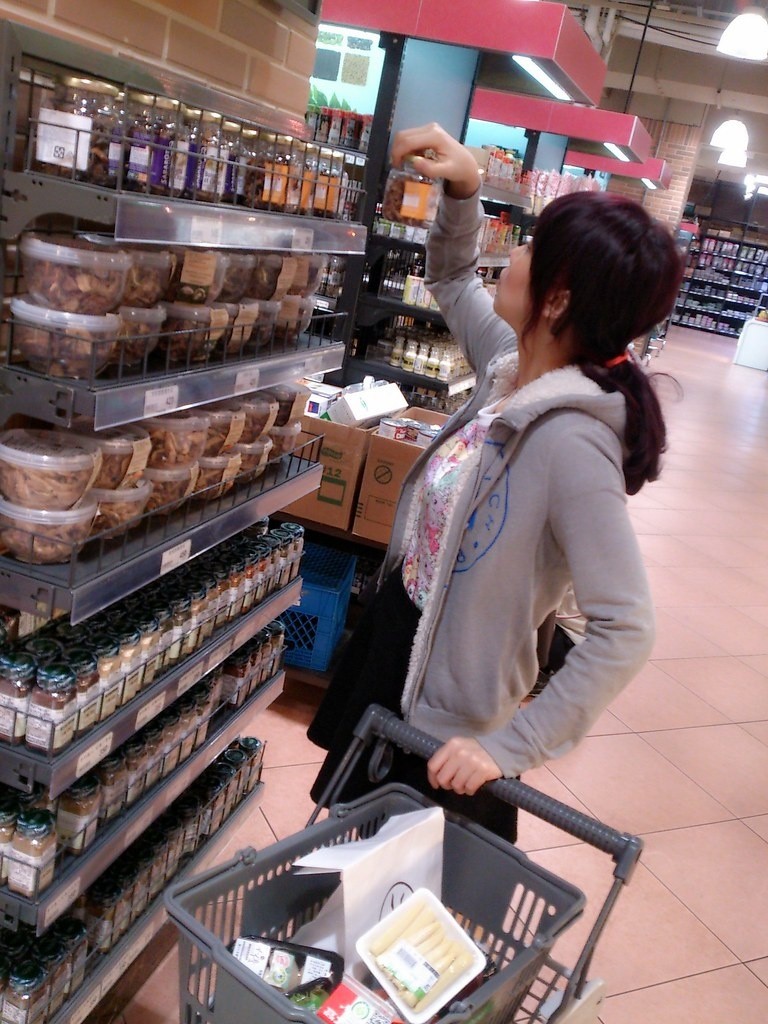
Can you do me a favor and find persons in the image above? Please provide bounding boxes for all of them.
[307,123,683,844]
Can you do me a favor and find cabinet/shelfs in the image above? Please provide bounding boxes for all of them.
[669,232,768,338]
[0,18,369,1024]
[344,168,532,398]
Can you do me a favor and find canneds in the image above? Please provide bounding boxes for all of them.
[511,225,521,244]
[29,76,376,223]
[378,417,441,448]
[0,515,305,1024]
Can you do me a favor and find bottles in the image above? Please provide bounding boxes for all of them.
[378,326,476,382]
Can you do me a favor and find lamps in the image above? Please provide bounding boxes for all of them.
[709,118,748,152]
[716,13,768,60]
[717,150,747,167]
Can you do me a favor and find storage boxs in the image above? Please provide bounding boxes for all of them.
[351,407,451,545]
[317,971,396,1024]
[298,379,343,418]
[355,886,487,1024]
[707,229,719,236]
[274,542,358,671]
[319,382,409,429]
[278,408,406,532]
[719,230,731,238]
[227,937,345,988]
[163,784,587,1024]
